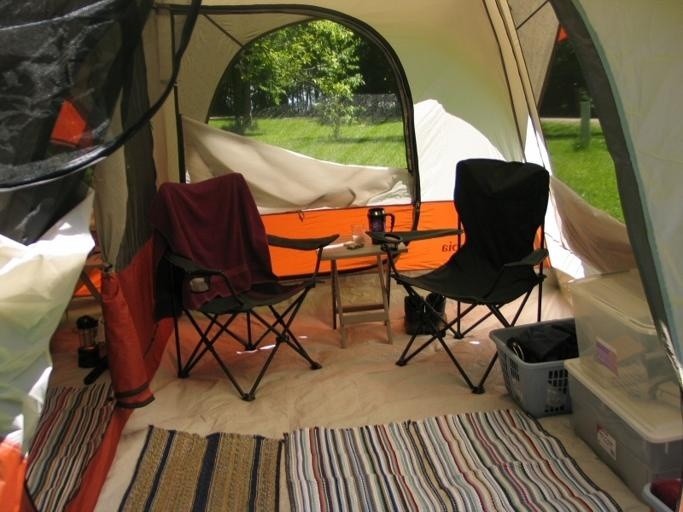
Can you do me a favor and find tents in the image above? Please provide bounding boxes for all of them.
[0,1,683,511]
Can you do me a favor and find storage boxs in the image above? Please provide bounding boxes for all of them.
[563,359,683,502]
[569,267,672,403]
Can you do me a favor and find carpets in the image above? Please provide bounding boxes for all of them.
[23,381,116,512]
[118,425,282,511]
[283,406,624,512]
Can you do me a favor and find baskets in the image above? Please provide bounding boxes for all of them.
[488,317,578,418]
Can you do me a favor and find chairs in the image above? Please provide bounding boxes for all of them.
[148,171,340,402]
[383,159,550,394]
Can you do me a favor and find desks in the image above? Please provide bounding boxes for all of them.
[315,237,408,349]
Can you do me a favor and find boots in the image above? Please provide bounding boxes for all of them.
[404,292,447,336]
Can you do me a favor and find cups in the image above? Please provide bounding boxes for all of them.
[351,223,365,243]
[367,209,395,244]
